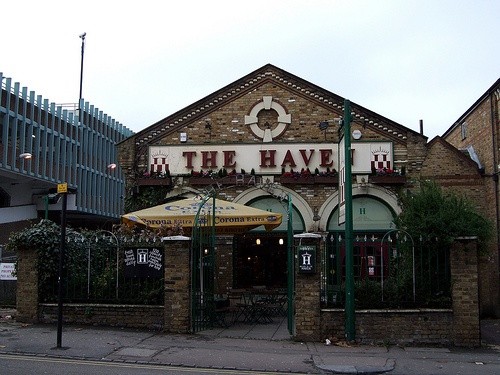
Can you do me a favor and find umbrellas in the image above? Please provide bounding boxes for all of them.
[122,194,282,321]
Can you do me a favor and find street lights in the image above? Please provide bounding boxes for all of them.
[57,183,78,349]
[77,32,88,109]
[105,164,117,233]
